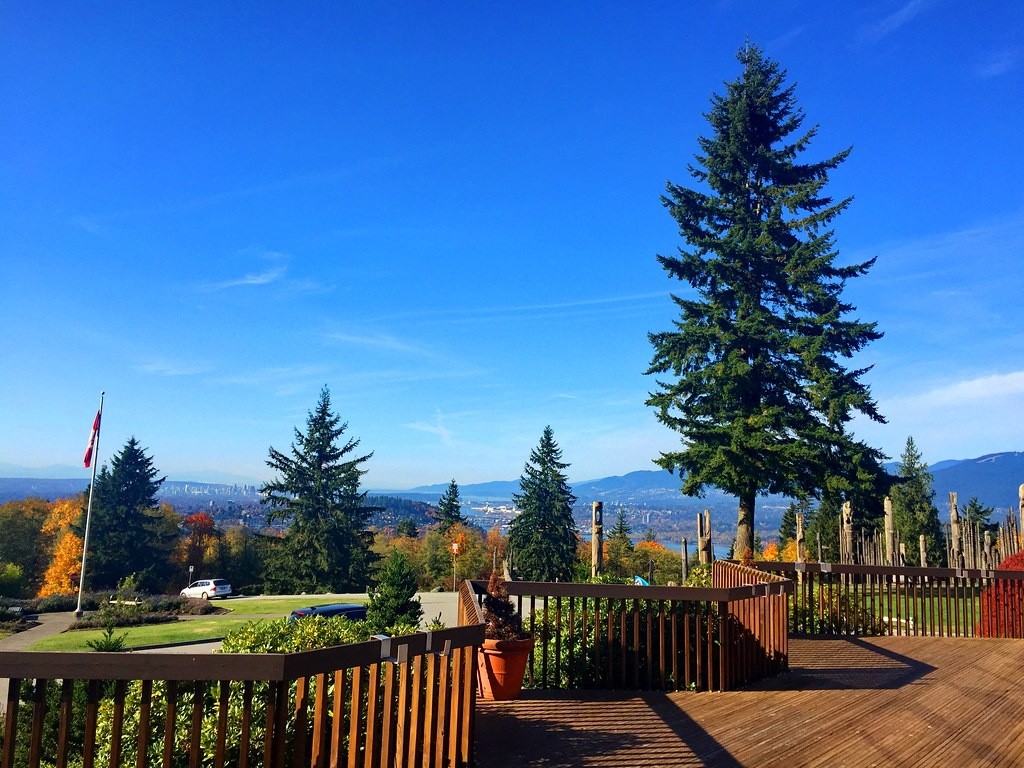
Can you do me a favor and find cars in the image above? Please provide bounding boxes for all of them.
[180,579,232,601]
[288,603,367,626]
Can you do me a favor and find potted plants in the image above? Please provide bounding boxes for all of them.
[476,525,536,701]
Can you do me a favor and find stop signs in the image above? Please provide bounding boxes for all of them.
[70,573,78,581]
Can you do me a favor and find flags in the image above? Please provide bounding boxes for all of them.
[83,410,100,468]
